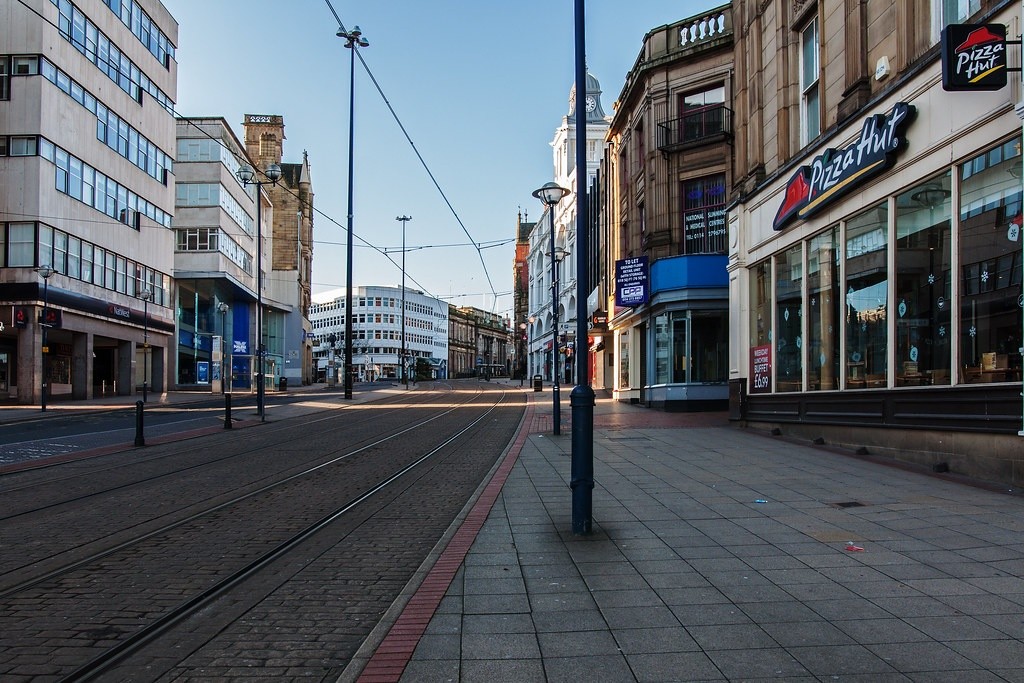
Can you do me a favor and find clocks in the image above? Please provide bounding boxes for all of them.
[585,96,597,113]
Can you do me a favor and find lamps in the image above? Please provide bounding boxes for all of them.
[1005,161,1023,181]
[910,183,951,210]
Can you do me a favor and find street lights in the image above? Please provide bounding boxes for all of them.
[218,303,231,395]
[142,288,156,402]
[533,181,570,435]
[519,323,527,386]
[529,315,534,388]
[396,215,414,385]
[544,247,572,324]
[337,24,370,400]
[235,162,284,417]
[33,265,59,412]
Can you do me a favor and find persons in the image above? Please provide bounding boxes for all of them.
[1007,335,1019,354]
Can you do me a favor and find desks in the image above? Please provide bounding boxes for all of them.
[846,379,887,388]
[966,367,1022,383]
[896,376,933,386]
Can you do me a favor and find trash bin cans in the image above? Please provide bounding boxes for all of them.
[534,374,543,392]
[279,377,287,391]
